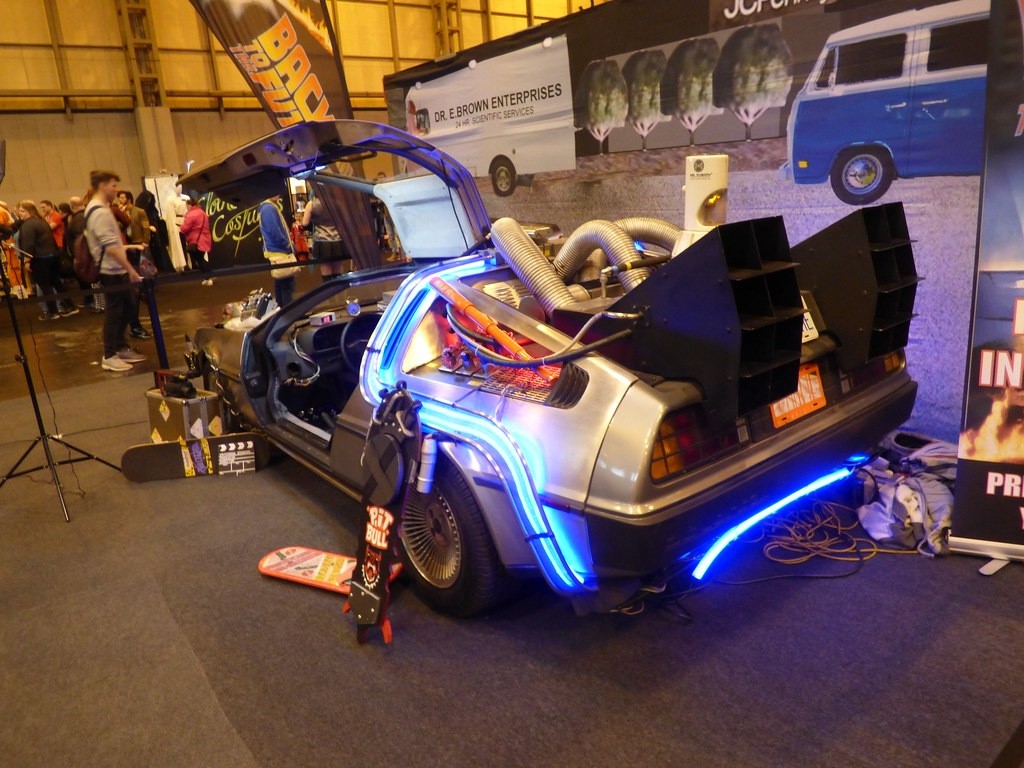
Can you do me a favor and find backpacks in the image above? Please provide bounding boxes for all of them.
[72,205,107,284]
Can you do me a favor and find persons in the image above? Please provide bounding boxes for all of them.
[258,189,302,305]
[302,188,351,285]
[84,169,149,371]
[289,211,309,267]
[0,189,171,322]
[181,199,216,287]
[366,171,404,263]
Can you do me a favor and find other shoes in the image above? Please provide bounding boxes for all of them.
[91,306,104,314]
[201,277,216,286]
[129,326,151,339]
[60,305,80,317]
[38,312,60,320]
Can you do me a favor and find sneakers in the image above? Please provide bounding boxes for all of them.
[101,348,146,372]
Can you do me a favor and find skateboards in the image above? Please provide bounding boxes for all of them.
[343,380,423,646]
[121,431,270,482]
[257,544,403,595]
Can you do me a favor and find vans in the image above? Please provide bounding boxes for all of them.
[776,0,990,205]
[401,35,577,198]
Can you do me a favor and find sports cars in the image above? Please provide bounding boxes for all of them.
[170,117,920,623]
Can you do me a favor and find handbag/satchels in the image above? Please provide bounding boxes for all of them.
[27,255,48,284]
[185,244,197,253]
